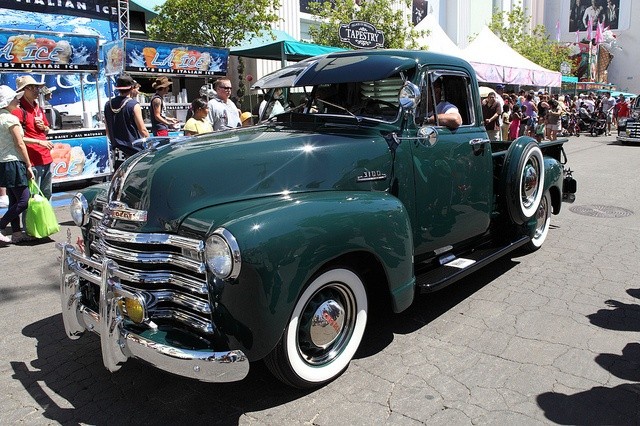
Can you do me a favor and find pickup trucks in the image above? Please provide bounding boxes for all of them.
[54,51,566,391]
[618,97,640,142]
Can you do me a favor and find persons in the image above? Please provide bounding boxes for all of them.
[258,87,285,121]
[149,76,179,137]
[0,84,35,243]
[103,71,150,175]
[361,72,462,129]
[203,75,242,133]
[130,80,141,97]
[238,110,260,129]
[183,98,213,139]
[11,74,54,240]
[482,83,637,144]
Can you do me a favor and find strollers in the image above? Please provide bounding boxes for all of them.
[592,110,611,137]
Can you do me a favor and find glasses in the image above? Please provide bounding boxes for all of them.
[218,86,233,90]
[25,85,39,90]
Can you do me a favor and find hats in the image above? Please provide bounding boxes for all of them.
[0,84,26,110]
[240,110,258,122]
[15,74,47,94]
[151,76,174,90]
[112,75,138,90]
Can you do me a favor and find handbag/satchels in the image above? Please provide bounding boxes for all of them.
[25,176,60,239]
